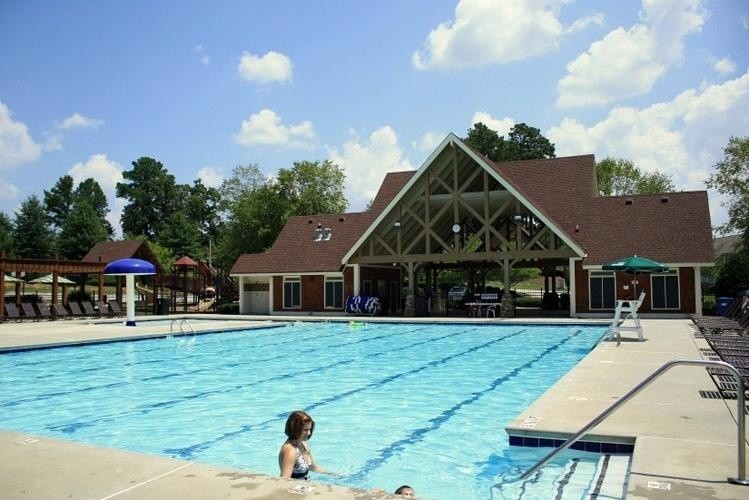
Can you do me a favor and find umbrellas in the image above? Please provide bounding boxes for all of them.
[4,274,26,284]
[601,254,669,300]
[28,274,77,299]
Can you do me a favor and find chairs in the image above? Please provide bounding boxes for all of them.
[692,295,749,416]
[0,300,126,324]
[612,291,646,340]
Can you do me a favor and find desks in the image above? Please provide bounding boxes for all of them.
[465,303,502,317]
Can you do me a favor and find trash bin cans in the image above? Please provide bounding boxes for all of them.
[717,297,733,315]
[157,298,169,315]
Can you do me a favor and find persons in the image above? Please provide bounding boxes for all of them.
[278,411,344,480]
[394,485,415,498]
